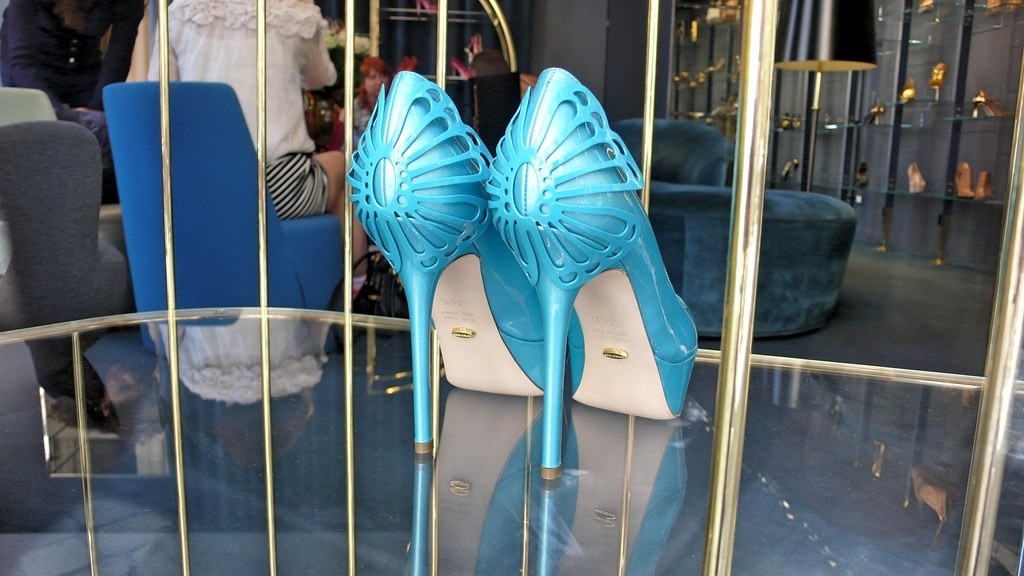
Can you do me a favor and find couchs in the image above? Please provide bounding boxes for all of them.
[608,117,859,338]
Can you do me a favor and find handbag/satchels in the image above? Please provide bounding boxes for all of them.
[324,253,412,340]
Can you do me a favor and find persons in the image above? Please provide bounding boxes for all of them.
[149,0,368,302]
[25,307,343,468]
[1,0,537,155]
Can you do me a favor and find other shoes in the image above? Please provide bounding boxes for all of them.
[917,0,935,15]
[782,158,798,181]
[672,0,742,124]
[984,0,1022,16]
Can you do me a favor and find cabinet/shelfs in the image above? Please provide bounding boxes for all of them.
[380,6,488,81]
[667,0,1023,266]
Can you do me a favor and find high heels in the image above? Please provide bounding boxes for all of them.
[929,62,949,91]
[908,162,925,193]
[856,162,869,189]
[344,68,699,482]
[955,160,995,201]
[779,104,887,131]
[899,77,917,105]
[973,90,1016,118]
[405,383,690,576]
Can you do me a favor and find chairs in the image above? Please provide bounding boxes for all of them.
[0,86,128,532]
[100,82,346,368]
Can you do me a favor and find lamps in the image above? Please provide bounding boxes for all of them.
[774,0,877,71]
